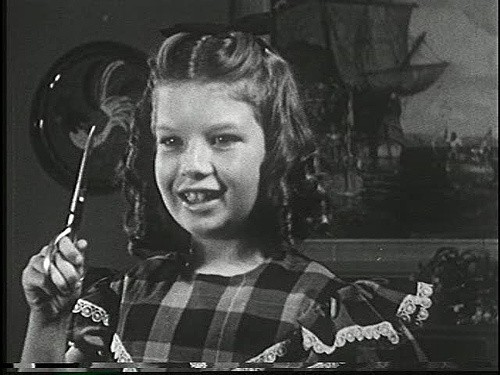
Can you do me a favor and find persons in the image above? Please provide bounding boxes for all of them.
[16,29,435,374]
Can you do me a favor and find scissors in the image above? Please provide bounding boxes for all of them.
[33,115,97,288]
